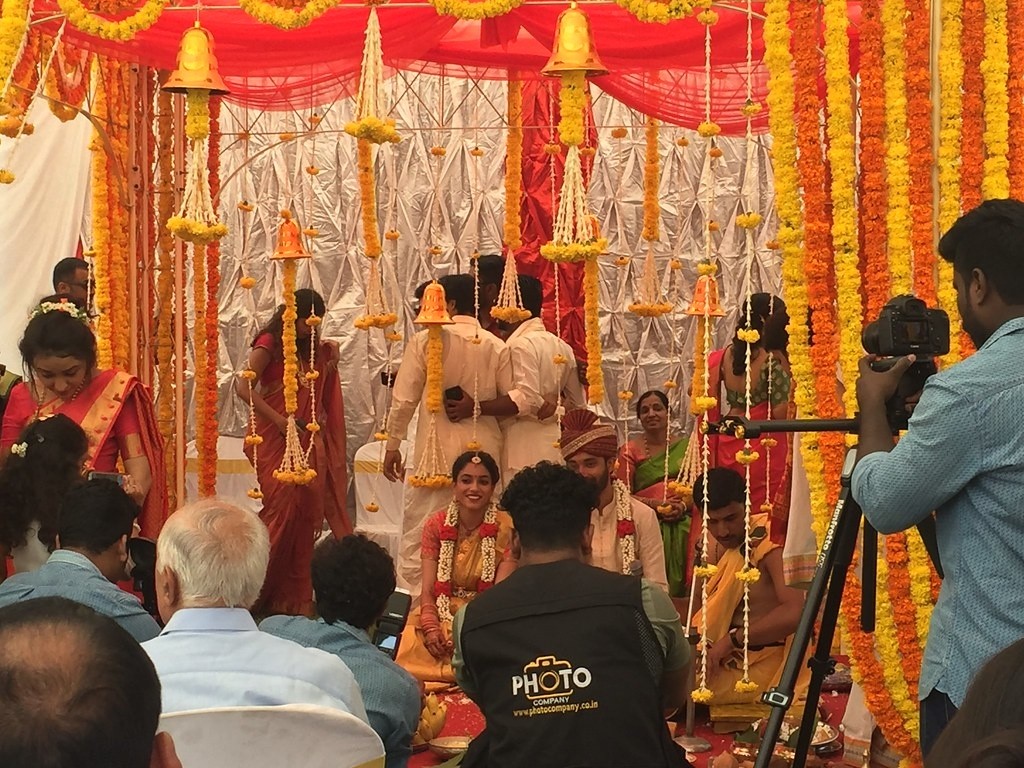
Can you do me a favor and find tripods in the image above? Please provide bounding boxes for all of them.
[753,355,945,768]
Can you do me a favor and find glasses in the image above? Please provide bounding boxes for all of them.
[69,279,95,290]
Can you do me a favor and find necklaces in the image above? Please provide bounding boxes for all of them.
[715,543,721,563]
[31,378,86,423]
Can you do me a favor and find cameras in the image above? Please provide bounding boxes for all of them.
[862,293,950,356]
[373,591,412,661]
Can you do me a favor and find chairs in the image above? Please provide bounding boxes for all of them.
[186,435,264,514]
[353,440,412,592]
[155,703,386,768]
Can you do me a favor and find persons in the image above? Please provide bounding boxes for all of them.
[616,390,701,625]
[394,448,515,683]
[855,199,1024,768]
[0,478,160,641]
[36,257,96,312]
[452,459,695,768]
[0,366,21,434]
[383,254,567,612]
[138,499,371,768]
[0,413,89,584]
[707,293,796,549]
[686,466,805,706]
[0,293,168,581]
[237,288,354,627]
[0,597,180,768]
[558,409,669,657]
[259,533,421,768]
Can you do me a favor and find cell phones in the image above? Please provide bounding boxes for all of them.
[446,386,463,407]
[381,372,396,388]
[88,472,124,486]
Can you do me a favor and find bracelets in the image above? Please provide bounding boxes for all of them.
[729,626,742,649]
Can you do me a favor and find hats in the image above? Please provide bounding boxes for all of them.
[559,409,618,458]
